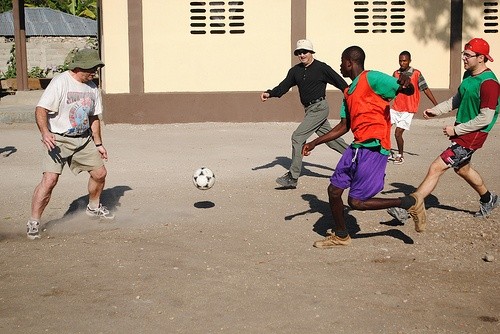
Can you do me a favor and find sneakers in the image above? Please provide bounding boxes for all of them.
[276,171,298,186]
[406,192,427,232]
[313,232,351,249]
[474,193,500,217]
[26,219,39,238]
[86,202,115,219]
[387,207,409,226]
[393,154,405,165]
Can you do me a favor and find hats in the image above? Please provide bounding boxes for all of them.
[294,39,316,56]
[465,38,494,62]
[67,48,105,70]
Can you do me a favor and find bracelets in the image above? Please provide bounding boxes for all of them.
[96,144,102,147]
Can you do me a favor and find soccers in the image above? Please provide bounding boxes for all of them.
[192,167,215,190]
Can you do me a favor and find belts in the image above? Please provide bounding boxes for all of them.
[48,130,83,138]
[305,96,325,107]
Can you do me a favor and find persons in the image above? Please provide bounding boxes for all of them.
[387,38,499,222]
[381,49,444,165]
[299,45,426,249]
[259,37,355,188]
[24,48,116,239]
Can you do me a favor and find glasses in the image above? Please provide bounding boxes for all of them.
[461,51,480,58]
[298,50,309,55]
